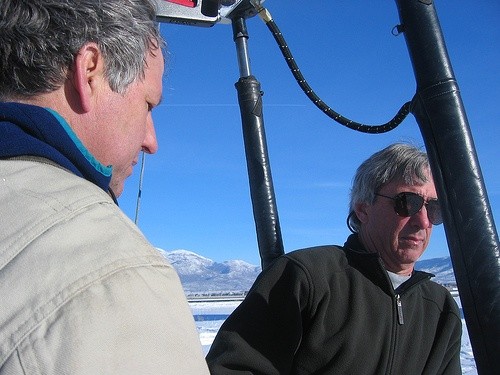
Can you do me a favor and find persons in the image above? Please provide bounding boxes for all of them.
[0,0,213,375]
[205,143,463,375]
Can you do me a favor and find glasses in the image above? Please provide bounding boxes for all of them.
[372,191,443,226]
